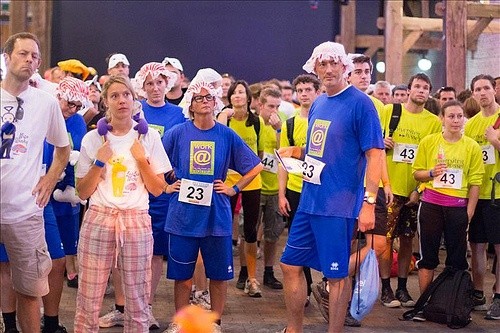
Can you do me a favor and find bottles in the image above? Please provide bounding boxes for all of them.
[437,145,445,164]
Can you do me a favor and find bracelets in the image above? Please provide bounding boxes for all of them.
[276,129,281,132]
[429,169,434,178]
[92,159,105,167]
[165,184,169,193]
[365,191,377,198]
[233,185,240,193]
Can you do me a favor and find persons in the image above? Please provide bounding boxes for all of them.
[235,41,500,333]
[0,32,263,333]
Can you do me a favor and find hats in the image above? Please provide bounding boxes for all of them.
[132,61,178,100]
[346,53,373,75]
[163,57,183,75]
[177,68,226,118]
[58,59,90,81]
[88,67,97,77]
[302,41,355,78]
[84,75,101,92]
[57,76,95,110]
[109,54,130,71]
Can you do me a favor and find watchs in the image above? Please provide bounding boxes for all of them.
[363,196,376,204]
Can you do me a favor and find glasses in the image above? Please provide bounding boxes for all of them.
[67,101,86,112]
[15,97,24,120]
[192,94,214,103]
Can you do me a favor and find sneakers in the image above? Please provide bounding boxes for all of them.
[311,281,329,324]
[484,298,500,320]
[395,287,416,306]
[380,289,401,307]
[472,296,488,310]
[345,303,361,327]
[1,237,310,332]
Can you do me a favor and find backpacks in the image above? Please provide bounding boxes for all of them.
[349,229,379,320]
[402,265,474,330]
